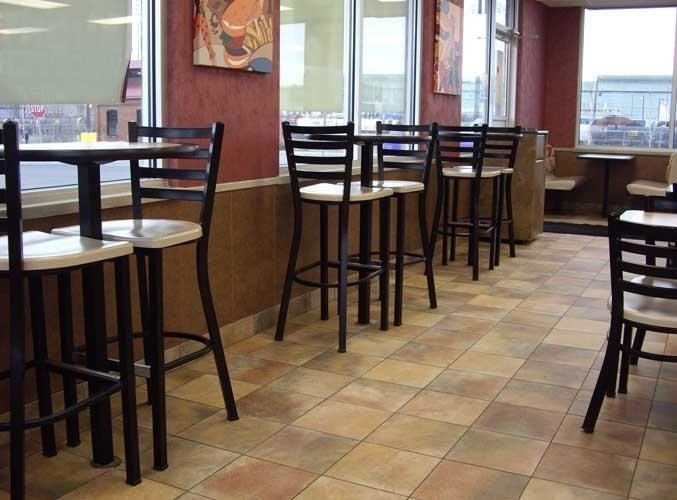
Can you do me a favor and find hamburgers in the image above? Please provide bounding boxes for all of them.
[219,0,263,69]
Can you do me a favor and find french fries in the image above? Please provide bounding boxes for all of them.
[242,14,273,55]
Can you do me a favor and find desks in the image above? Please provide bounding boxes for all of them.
[1,141,199,467]
[440,133,525,264]
[304,132,432,325]
[577,153,634,217]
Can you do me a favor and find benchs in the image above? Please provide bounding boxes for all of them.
[627,151,677,211]
[544,147,582,214]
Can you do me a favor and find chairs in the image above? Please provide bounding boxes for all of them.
[451,123,522,259]
[273,120,394,353]
[424,122,501,280]
[1,117,144,499]
[581,215,676,433]
[51,121,240,469]
[338,120,438,326]
